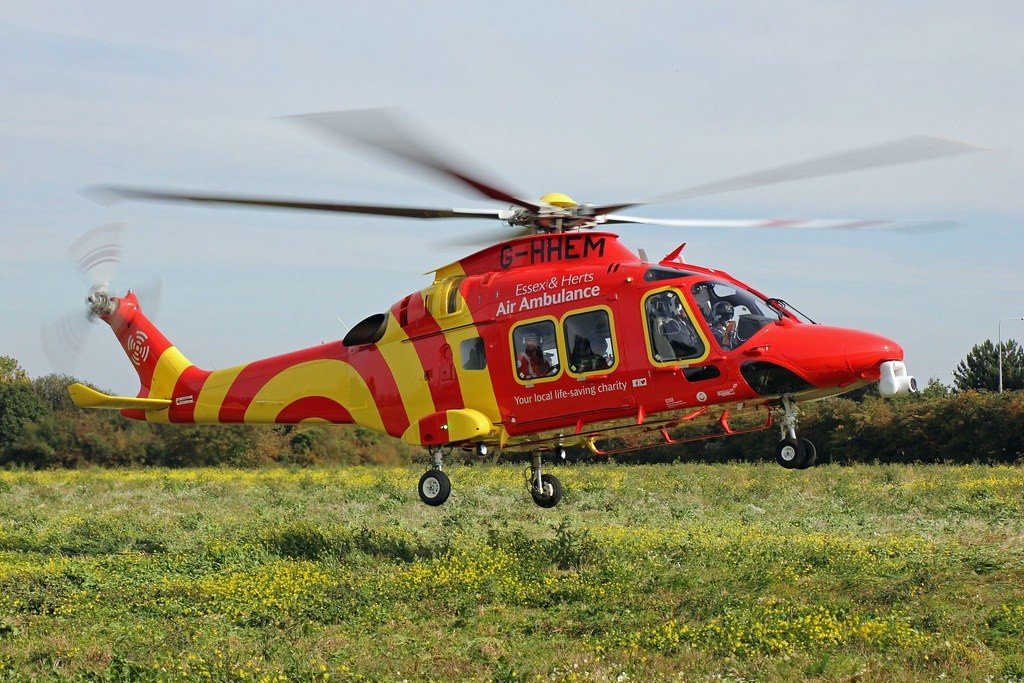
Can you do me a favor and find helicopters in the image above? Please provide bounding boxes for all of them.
[68,107,980,509]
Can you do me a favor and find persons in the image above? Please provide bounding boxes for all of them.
[706,299,736,354]
[574,336,613,375]
[663,295,725,362]
[515,328,554,382]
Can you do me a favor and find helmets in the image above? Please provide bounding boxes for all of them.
[712,301,734,322]
[522,327,544,345]
[668,296,684,315]
